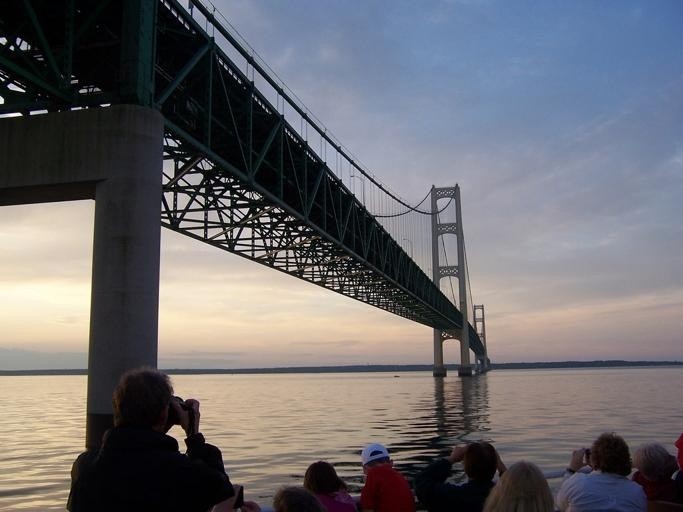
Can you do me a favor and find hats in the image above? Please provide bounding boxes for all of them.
[362,443,390,465]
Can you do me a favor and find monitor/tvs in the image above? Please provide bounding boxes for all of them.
[585,448,591,458]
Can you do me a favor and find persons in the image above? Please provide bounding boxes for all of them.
[67,368,235,512]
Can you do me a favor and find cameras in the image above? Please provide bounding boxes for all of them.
[167,395,191,425]
